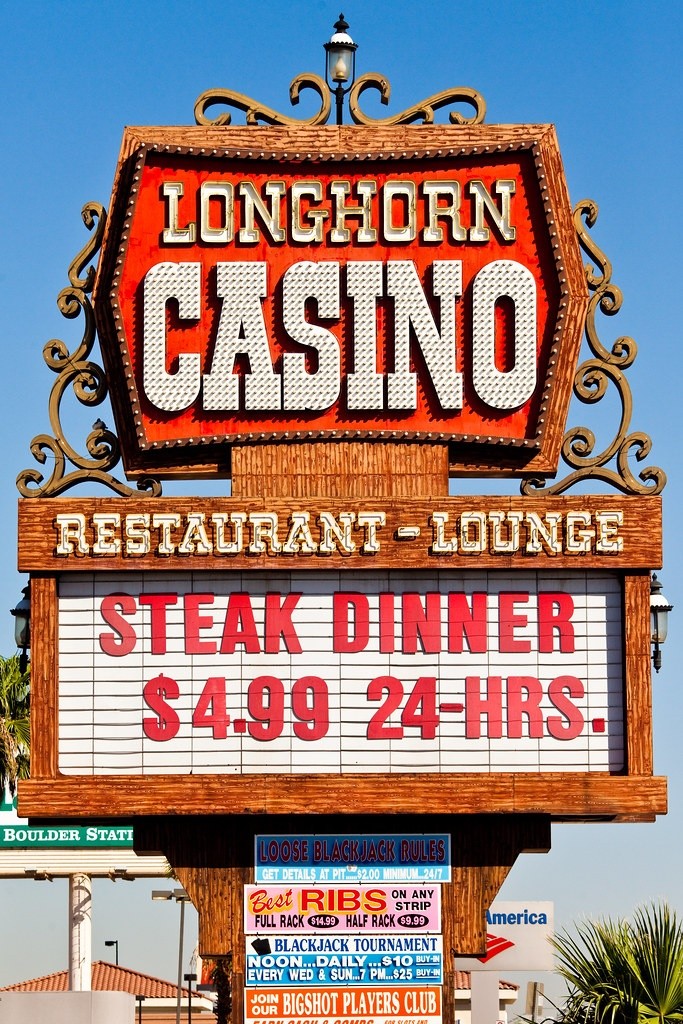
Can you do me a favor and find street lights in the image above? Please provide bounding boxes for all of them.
[184,973,197,1024]
[136,996,145,1024]
[151,887,192,1024]
[105,940,119,965]
